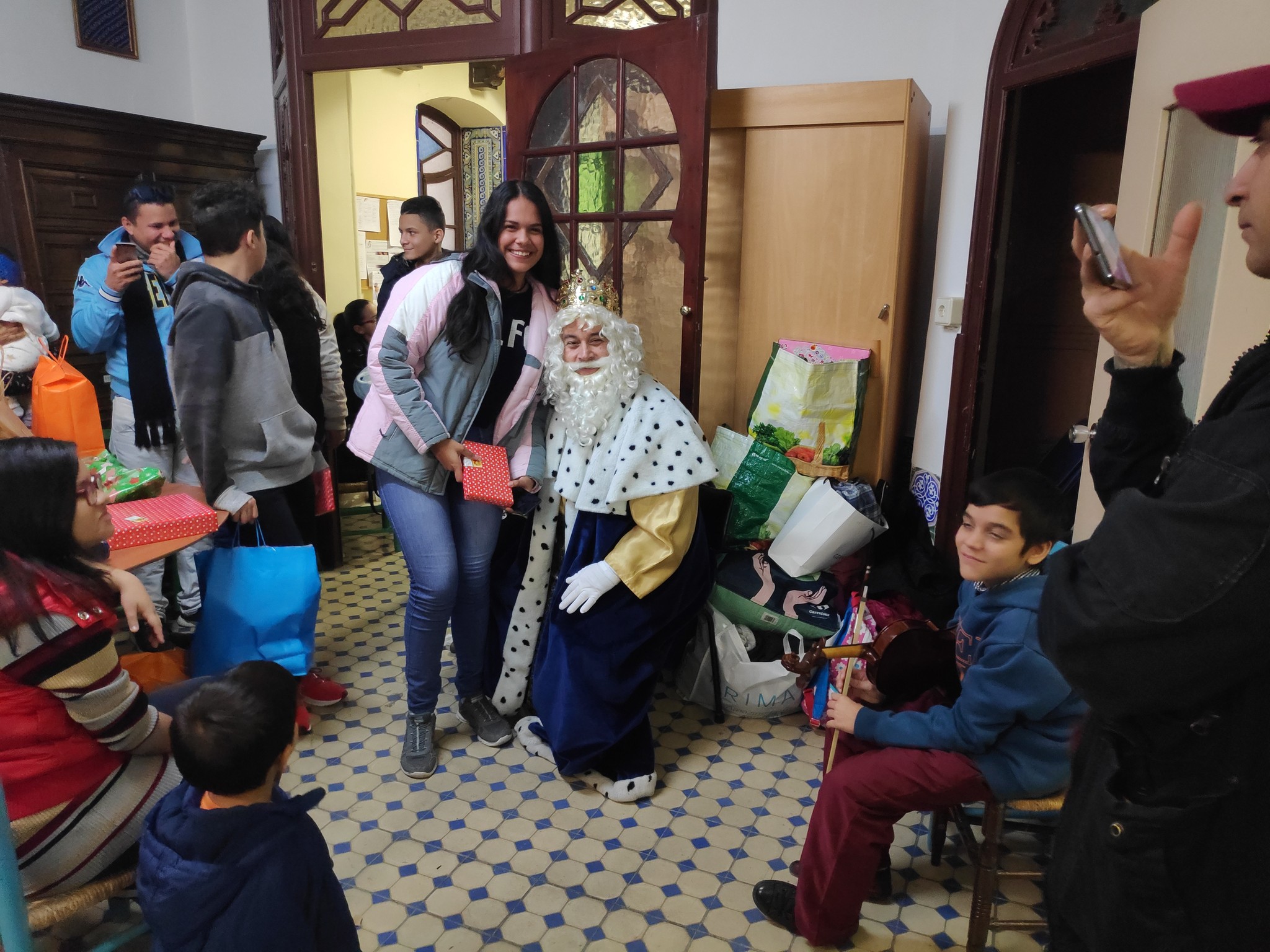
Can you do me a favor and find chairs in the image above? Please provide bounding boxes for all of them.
[0,786,149,952]
[336,441,401,552]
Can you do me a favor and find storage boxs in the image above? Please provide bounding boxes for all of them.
[106,493,219,550]
[461,440,514,507]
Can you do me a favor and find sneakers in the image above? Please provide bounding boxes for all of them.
[456,694,513,747]
[293,701,314,735]
[298,667,348,708]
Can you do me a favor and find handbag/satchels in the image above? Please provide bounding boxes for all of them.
[766,477,890,577]
[184,512,321,678]
[31,334,107,459]
[745,340,873,483]
[0,344,36,438]
[676,598,819,719]
[707,426,815,539]
[708,549,839,639]
[800,592,922,729]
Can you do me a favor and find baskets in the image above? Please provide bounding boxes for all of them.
[785,420,849,480]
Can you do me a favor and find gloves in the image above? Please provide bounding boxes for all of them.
[559,560,622,614]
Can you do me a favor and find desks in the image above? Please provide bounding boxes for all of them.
[105,483,229,571]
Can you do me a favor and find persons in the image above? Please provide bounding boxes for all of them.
[486,269,721,802]
[1037,59,1270,952]
[1,179,447,735]
[751,467,1088,946]
[0,437,219,905]
[348,181,568,779]
[134,660,364,952]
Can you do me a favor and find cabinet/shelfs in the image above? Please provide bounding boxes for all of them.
[600,78,931,485]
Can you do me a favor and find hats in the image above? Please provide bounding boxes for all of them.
[0,254,21,284]
[1174,64,1270,136]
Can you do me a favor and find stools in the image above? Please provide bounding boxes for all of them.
[929,794,1065,952]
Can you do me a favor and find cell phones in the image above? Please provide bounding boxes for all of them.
[1074,202,1134,290]
[115,243,139,276]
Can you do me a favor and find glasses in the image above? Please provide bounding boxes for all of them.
[359,315,377,326]
[75,472,105,506]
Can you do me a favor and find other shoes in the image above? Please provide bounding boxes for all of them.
[790,860,895,905]
[399,709,439,779]
[130,619,171,654]
[177,606,205,627]
[752,879,810,937]
[287,787,326,815]
